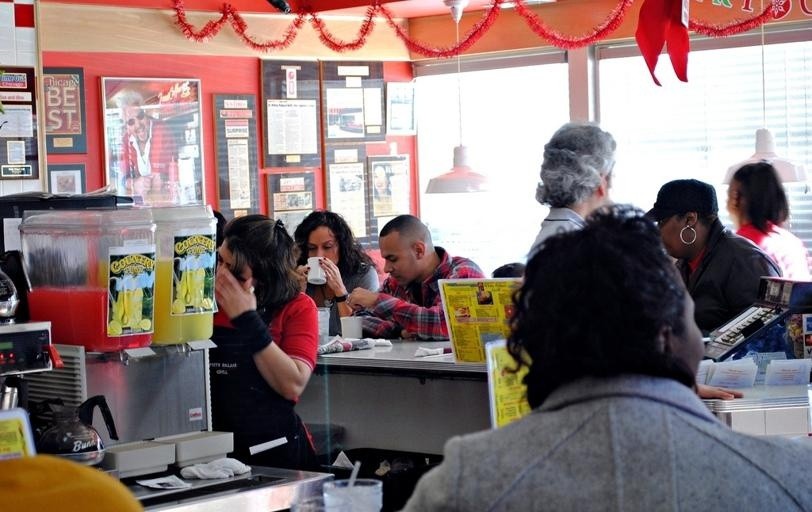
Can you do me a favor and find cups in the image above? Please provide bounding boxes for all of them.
[339,316,364,339]
[318,308,331,344]
[306,256,327,286]
[323,478,384,512]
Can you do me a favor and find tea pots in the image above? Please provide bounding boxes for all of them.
[36,395,118,467]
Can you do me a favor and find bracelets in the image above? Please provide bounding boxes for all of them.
[332,295,350,304]
[229,311,273,355]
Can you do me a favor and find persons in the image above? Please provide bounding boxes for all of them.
[373,165,394,216]
[114,89,178,207]
[528,122,617,261]
[345,214,487,342]
[397,205,812,511]
[642,179,784,337]
[294,212,380,336]
[208,216,320,473]
[728,162,811,283]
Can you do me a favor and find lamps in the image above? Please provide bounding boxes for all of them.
[722,1,806,184]
[424,0,489,194]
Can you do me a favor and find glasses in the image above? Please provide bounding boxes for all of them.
[125,109,146,126]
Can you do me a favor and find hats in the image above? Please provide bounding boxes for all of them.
[644,179,719,222]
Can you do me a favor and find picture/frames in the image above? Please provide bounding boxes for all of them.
[258,57,320,167]
[386,81,417,135]
[48,164,86,196]
[100,77,206,207]
[0,66,40,182]
[367,154,411,218]
[43,66,86,154]
[325,144,372,250]
[264,172,315,242]
[212,93,260,222]
[321,61,387,143]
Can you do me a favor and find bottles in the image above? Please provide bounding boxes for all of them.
[168,154,179,182]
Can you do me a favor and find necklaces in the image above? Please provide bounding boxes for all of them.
[319,284,336,308]
